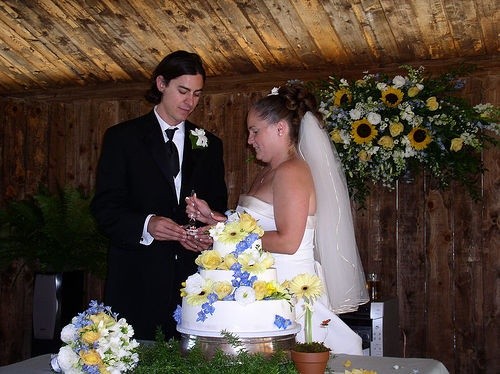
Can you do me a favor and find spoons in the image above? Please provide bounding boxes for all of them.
[188,189,195,225]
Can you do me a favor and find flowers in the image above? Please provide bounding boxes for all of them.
[189,128,209,150]
[49,300,140,374]
[173,210,292,329]
[289,273,331,353]
[302,64,500,211]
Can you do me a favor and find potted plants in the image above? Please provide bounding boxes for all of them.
[0,180,108,357]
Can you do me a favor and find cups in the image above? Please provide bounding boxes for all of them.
[367,274,382,302]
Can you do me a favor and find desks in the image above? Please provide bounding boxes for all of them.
[0,339,449,374]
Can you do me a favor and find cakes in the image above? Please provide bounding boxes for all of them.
[179,232,297,333]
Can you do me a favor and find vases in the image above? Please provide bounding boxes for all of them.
[291,349,329,374]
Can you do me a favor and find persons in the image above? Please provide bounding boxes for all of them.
[184,85,371,358]
[87,48,230,344]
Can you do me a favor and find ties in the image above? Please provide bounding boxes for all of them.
[164,127,180,177]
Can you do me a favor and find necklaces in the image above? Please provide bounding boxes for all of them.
[259,169,275,183]
